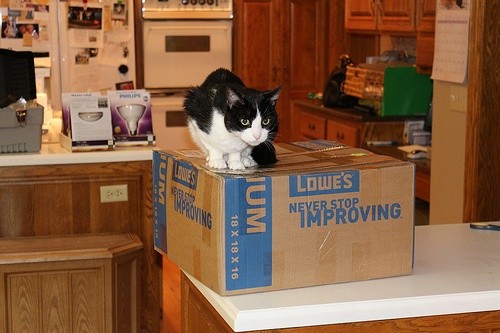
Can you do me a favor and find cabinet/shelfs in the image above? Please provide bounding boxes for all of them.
[289,99,425,162]
[0,141,164,333]
[345,0,436,74]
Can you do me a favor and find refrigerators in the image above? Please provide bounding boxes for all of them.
[0,0,136,111]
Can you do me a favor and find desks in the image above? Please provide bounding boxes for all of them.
[161,220,500,333]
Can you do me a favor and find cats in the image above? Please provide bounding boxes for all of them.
[182,68,283,171]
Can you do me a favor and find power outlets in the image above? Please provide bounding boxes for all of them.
[100,183,129,205]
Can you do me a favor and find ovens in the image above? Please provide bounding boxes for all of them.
[143,0,233,150]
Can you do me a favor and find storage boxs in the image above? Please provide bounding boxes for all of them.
[152,139,415,296]
